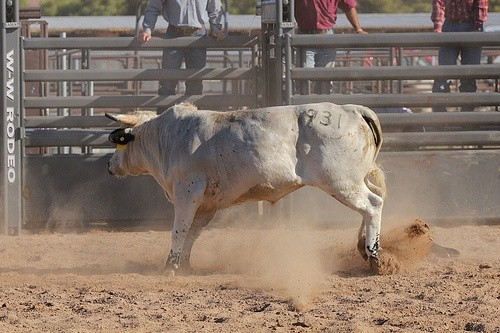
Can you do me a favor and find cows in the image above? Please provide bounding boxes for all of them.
[104,103,389,276]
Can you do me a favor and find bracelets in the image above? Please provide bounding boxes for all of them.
[356,28,363,32]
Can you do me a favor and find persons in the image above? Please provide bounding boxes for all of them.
[292,0,369,95]
[427,0,489,113]
[136,0,224,117]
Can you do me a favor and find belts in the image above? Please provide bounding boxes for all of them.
[446,19,473,24]
[169,25,204,36]
[298,28,332,34]
